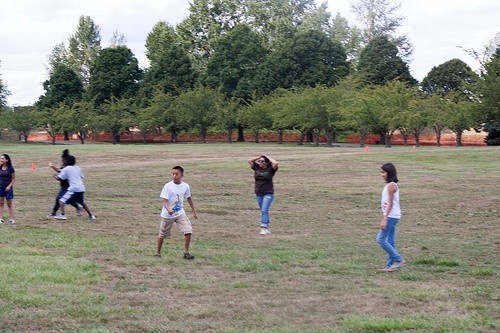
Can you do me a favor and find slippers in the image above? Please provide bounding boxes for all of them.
[390,263,406,271]
[382,266,397,271]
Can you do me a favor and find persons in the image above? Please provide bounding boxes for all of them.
[53,155,97,220]
[0,154,16,224]
[154,166,198,259]
[376,162,407,272]
[248,155,278,235]
[47,149,83,218]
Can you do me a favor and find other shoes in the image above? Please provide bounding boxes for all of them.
[0,218,4,223]
[90,214,98,219]
[77,204,83,215]
[183,251,196,259]
[259,228,266,234]
[265,228,270,233]
[154,252,162,257]
[9,218,15,223]
[54,214,67,219]
[47,213,56,217]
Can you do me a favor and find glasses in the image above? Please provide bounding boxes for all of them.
[258,160,268,163]
